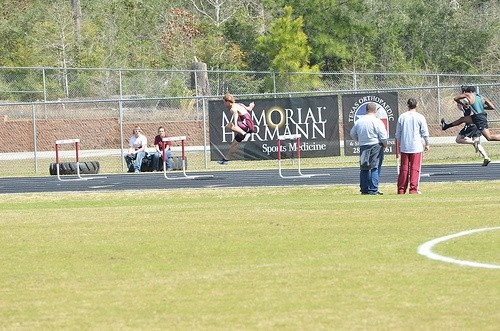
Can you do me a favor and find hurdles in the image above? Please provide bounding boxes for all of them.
[54,137,106,180]
[396,138,453,176]
[275,133,330,179]
[161,136,213,179]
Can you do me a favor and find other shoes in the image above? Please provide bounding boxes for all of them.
[473,141,481,153]
[482,158,490,166]
[440,117,448,131]
[216,157,228,165]
[241,133,251,143]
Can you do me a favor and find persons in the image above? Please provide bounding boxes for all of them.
[441,86,500,141]
[350,103,387,195]
[455,86,491,166]
[124,125,148,173]
[151,127,173,172]
[217,93,256,165]
[394,99,429,194]
